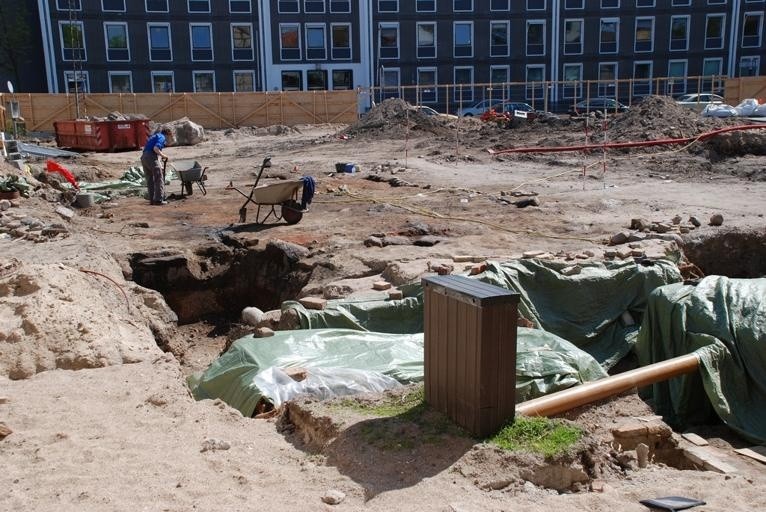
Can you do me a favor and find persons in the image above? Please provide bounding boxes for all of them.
[140,125,173,206]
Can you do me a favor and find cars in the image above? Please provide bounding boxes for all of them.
[456,98,510,118]
[568,98,629,117]
[494,31,507,43]
[472,102,552,120]
[675,93,725,115]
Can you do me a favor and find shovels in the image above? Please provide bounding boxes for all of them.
[162,157,168,201]
[239,157,271,223]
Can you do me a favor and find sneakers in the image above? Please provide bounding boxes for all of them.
[150,201,167,205]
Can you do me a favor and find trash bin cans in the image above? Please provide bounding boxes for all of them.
[421,275,521,442]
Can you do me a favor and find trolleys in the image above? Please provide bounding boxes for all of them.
[225,178,316,226]
[169,159,209,198]
